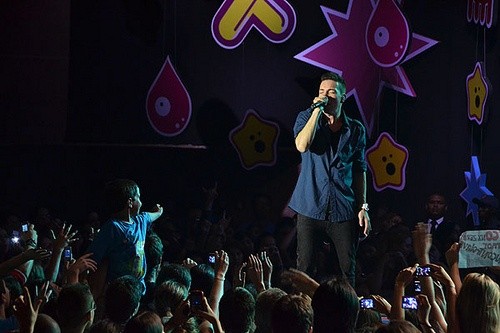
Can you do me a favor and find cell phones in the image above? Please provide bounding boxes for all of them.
[191,291,203,311]
[402,265,434,309]
[21,223,29,233]
[63,247,72,261]
[208,253,219,265]
[360,299,376,308]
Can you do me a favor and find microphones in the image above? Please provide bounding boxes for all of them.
[310,96,329,108]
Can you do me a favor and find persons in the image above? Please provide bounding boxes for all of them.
[0,192,500,333]
[288,73,371,287]
[89,180,163,298]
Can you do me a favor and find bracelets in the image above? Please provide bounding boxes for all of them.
[360,204,369,211]
[216,273,225,280]
